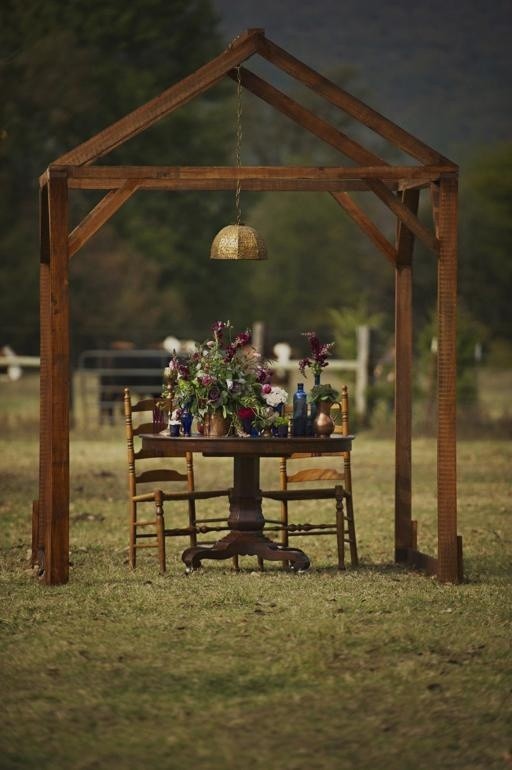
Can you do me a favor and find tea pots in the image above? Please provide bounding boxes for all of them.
[310,398,342,437]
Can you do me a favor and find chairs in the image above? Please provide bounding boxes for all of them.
[124,388,240,575]
[278,385,359,570]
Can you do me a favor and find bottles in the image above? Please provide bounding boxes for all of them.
[292,383,308,436]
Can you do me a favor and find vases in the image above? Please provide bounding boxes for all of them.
[204,411,233,438]
[313,400,341,435]
[307,376,320,435]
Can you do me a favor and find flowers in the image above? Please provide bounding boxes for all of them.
[156,319,288,437]
[299,331,335,378]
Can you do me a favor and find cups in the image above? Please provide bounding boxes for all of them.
[277,424,289,438]
[169,424,180,437]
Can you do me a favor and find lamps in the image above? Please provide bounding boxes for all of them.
[210,65,269,260]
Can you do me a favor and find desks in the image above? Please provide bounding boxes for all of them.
[138,433,356,575]
